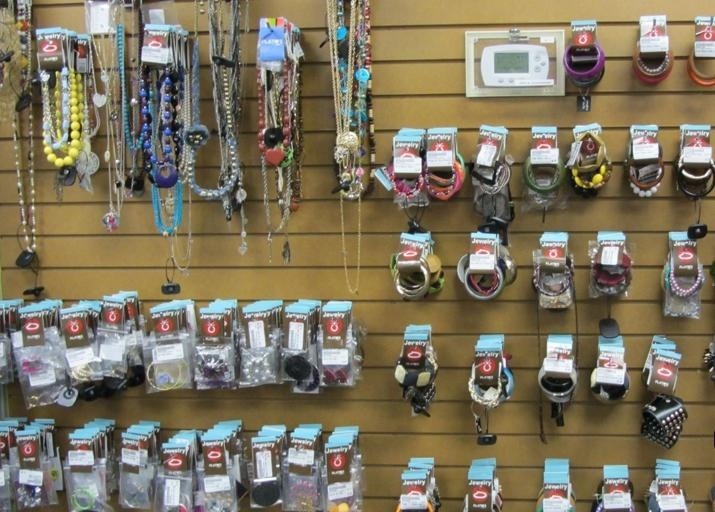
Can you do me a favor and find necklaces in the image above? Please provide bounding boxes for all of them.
[257,17,307,264]
[324,1,377,295]
[0,1,249,283]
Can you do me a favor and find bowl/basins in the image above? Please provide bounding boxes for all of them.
[324,1,377,295]
[257,17,307,264]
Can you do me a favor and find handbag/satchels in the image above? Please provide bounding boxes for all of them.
[563,41,605,86]
[687,44,715,86]
[635,42,674,84]
[0,299,359,511]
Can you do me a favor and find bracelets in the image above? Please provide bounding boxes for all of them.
[0,299,359,511]
[397,353,438,416]
[523,157,567,207]
[568,131,612,196]
[704,352,715,373]
[424,153,465,200]
[563,41,605,86]
[468,368,514,408]
[538,368,577,396]
[457,253,505,300]
[625,137,664,197]
[391,154,424,233]
[675,150,715,197]
[687,44,715,86]
[591,242,632,295]
[641,396,688,445]
[533,262,571,296]
[635,42,674,84]
[663,263,705,317]
[470,158,510,215]
[590,367,629,402]
[393,252,444,298]
